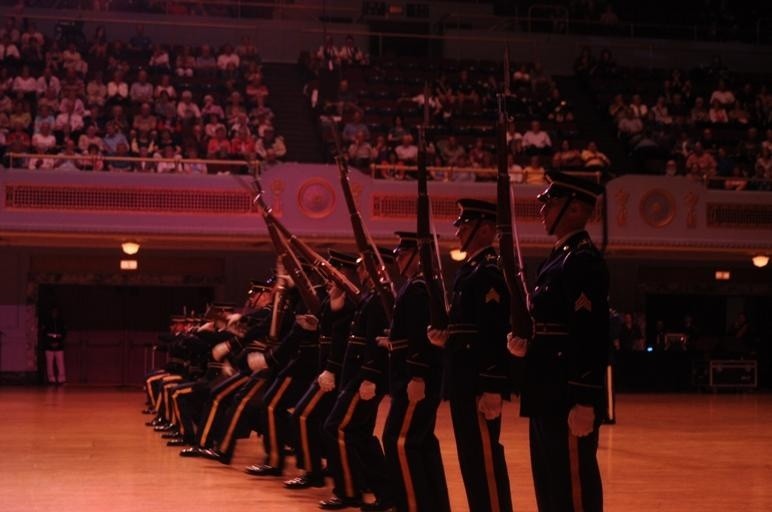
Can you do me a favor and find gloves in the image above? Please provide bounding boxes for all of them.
[567,403,597,438]
[478,392,502,420]
[406,379,426,402]
[427,325,450,347]
[212,340,233,376]
[507,332,528,357]
[359,380,376,400]
[247,352,269,373]
[318,370,336,391]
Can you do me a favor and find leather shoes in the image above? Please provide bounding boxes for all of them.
[281,477,326,489]
[361,499,391,511]
[142,406,230,465]
[244,464,282,476]
[318,496,365,510]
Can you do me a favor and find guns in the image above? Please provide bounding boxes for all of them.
[242,182,359,298]
[492,82,538,342]
[325,115,398,329]
[416,78,450,332]
[238,162,324,318]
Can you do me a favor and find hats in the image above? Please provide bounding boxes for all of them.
[169,301,235,323]
[297,230,441,272]
[247,280,274,296]
[452,198,497,225]
[536,172,605,206]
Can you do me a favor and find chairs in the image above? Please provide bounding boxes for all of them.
[317,53,572,137]
